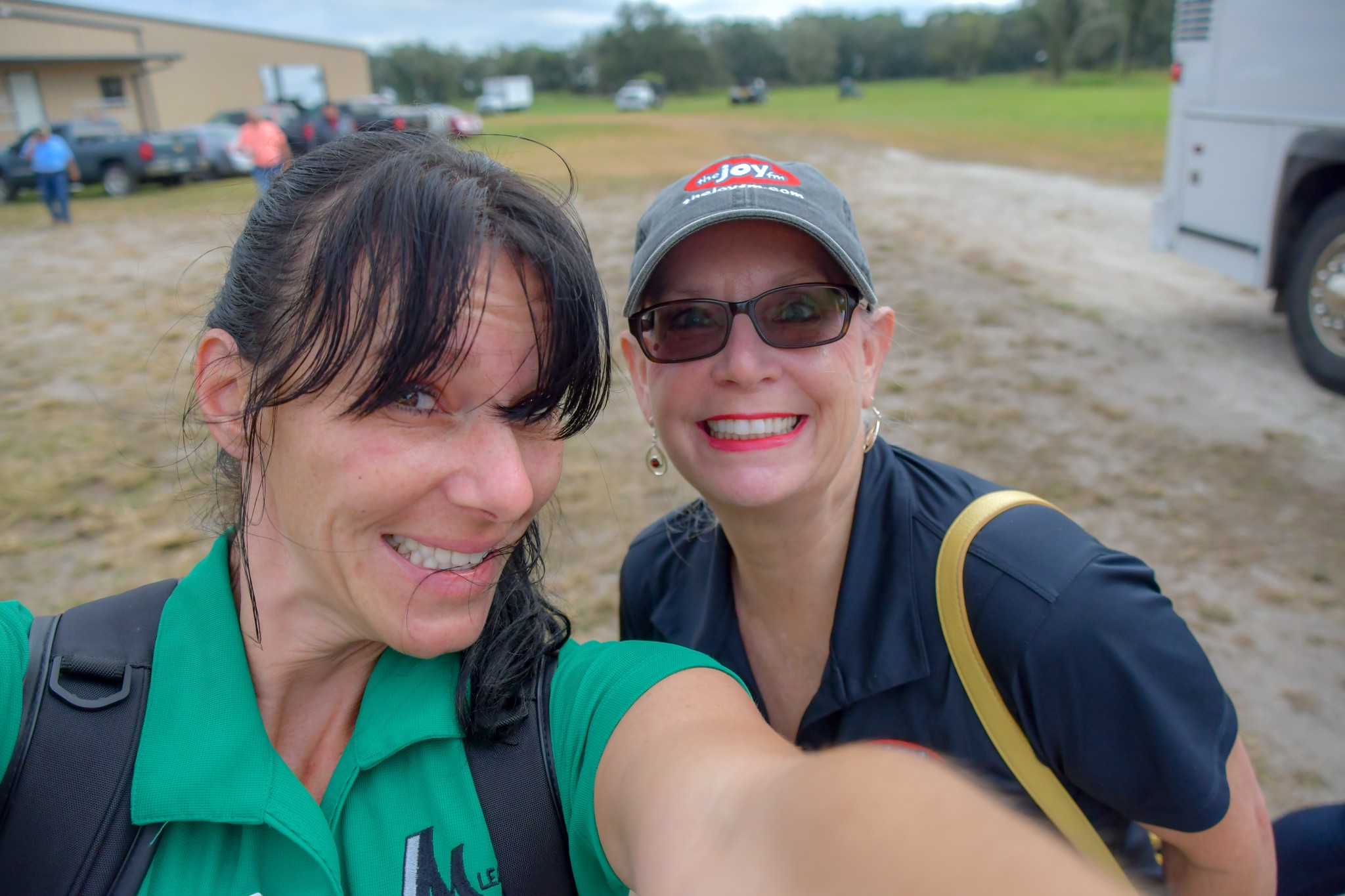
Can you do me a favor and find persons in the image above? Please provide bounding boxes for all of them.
[726,73,868,107]
[612,155,1277,896]
[310,101,355,147]
[238,106,292,199]
[0,132,1141,896]
[19,121,79,227]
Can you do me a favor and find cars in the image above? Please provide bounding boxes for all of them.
[181,97,482,202]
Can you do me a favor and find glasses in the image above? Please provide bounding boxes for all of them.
[628,282,859,362]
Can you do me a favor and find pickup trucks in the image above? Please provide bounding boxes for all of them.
[0,118,201,202]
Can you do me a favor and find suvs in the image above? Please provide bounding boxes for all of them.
[731,76,768,103]
[614,78,655,111]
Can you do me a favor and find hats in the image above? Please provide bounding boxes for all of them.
[621,154,877,316]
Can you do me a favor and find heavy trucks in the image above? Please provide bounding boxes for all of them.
[1149,1,1344,395]
